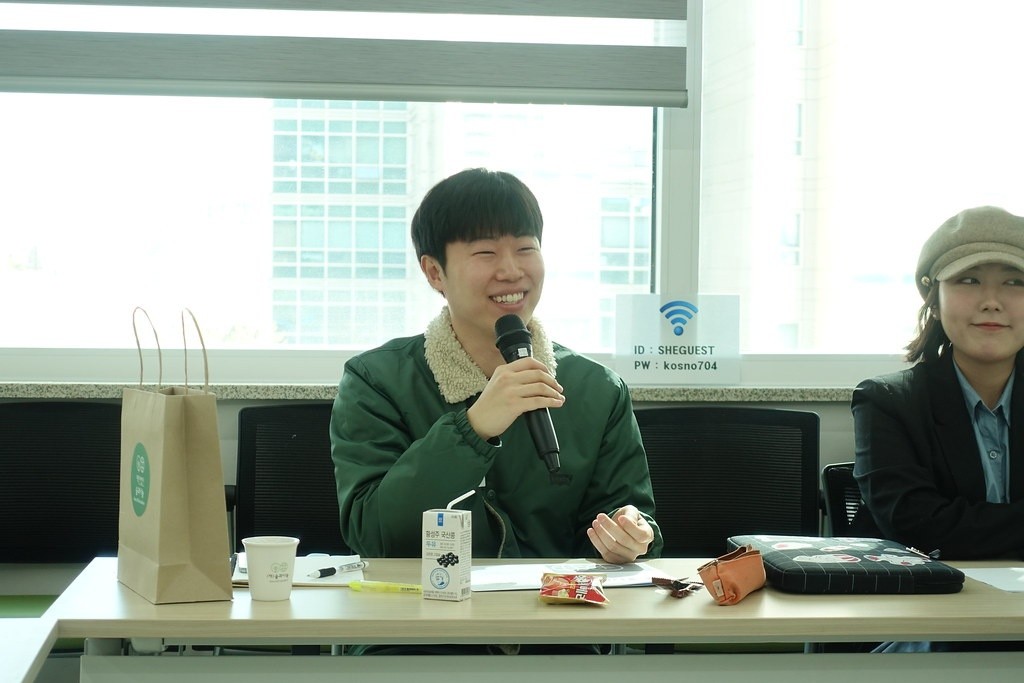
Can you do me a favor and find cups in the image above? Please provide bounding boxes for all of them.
[241,536,299,603]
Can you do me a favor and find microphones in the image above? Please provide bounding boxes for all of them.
[494,314,563,473]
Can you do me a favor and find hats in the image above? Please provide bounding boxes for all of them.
[916,206,1024,321]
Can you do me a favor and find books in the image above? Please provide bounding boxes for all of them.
[232,551,364,588]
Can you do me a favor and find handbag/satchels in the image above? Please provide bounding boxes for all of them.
[120,307,234,603]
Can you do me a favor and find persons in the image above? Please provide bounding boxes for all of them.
[329,167,664,657]
[824,205,1024,652]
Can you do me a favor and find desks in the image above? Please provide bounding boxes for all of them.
[49,553,1024,682]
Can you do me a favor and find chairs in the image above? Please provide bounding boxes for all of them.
[626,402,821,556]
[0,400,126,561]
[226,397,357,556]
